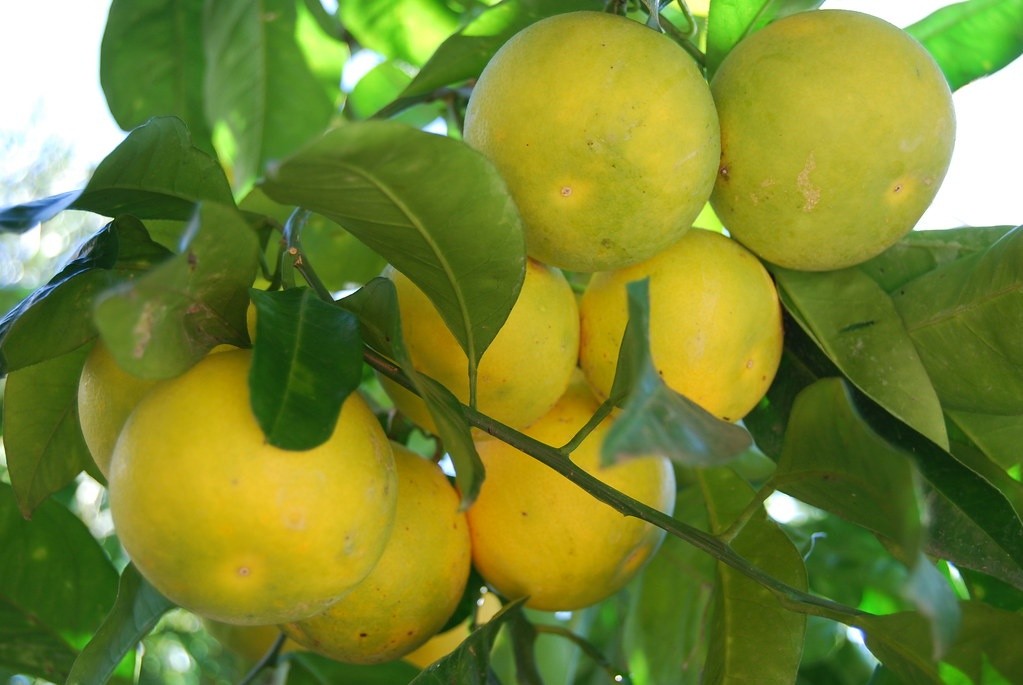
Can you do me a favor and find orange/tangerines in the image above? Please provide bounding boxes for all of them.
[76,8,957,669]
